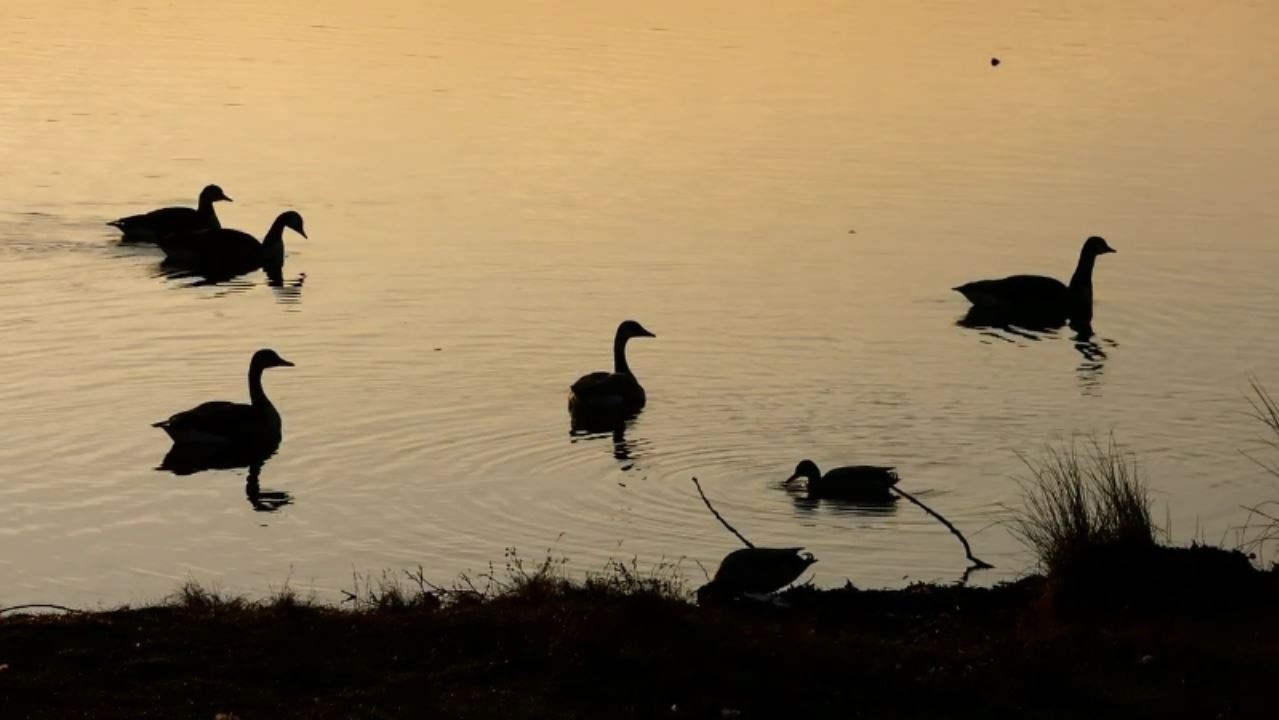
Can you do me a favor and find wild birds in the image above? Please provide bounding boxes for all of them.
[782,457,902,495]
[570,403,653,461]
[151,439,296,513]
[161,211,307,273]
[567,320,656,410]
[104,184,234,252]
[151,349,294,444]
[951,236,1119,312]
[791,493,900,511]
[952,304,1119,360]
